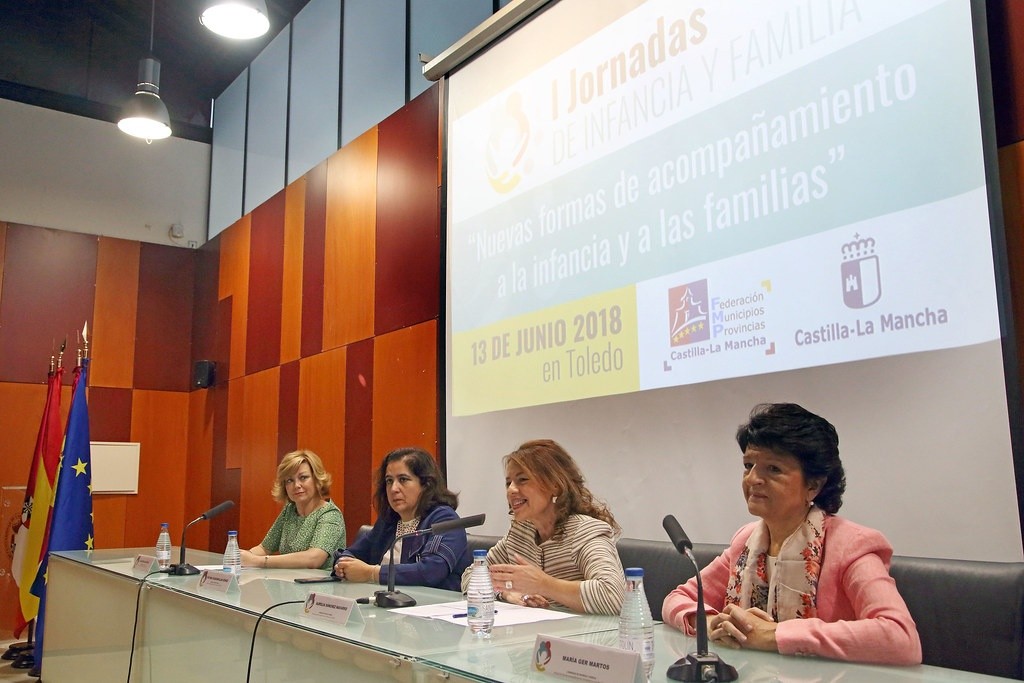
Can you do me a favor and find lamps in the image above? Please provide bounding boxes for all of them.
[117,0,172,141]
[200,0,271,40]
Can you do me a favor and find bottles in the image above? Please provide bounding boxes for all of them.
[156,523,171,569]
[618,568,655,682]
[467,549,495,639]
[222,531,241,585]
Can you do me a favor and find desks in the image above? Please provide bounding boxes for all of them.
[41,545,1024,683]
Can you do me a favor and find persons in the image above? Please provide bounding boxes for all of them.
[239,450,346,571]
[661,403,922,666]
[330,447,470,592]
[460,439,625,615]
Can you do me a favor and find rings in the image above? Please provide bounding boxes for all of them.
[522,595,529,601]
[506,581,512,589]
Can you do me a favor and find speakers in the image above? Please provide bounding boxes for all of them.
[195,360,214,388]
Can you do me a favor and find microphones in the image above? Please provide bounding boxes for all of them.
[374,514,486,608]
[663,515,739,683]
[168,500,234,575]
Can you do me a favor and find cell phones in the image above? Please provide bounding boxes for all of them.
[294,576,341,583]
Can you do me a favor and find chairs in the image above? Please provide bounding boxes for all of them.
[357,525,374,541]
[466,535,502,554]
[888,556,1024,682]
[616,538,730,622]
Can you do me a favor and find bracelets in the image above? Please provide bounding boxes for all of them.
[372,568,374,580]
[265,557,267,567]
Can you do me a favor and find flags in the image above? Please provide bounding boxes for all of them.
[11,358,94,673]
